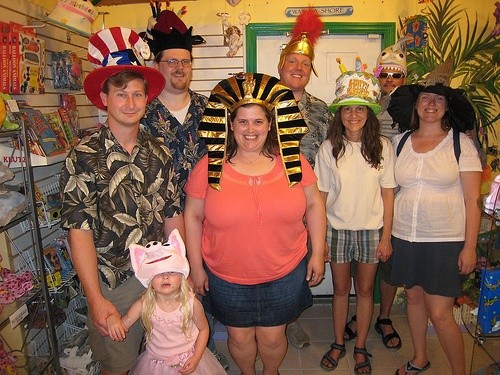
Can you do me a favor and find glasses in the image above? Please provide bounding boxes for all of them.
[379,72,404,79]
[161,58,192,67]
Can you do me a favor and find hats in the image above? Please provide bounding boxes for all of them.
[386,63,482,134]
[279,10,323,77]
[129,229,189,288]
[374,36,408,77]
[198,73,309,191]
[138,1,205,56]
[84,27,166,110]
[327,57,382,115]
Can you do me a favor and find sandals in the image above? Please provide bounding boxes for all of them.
[375,316,401,350]
[343,314,370,341]
[320,343,346,370]
[353,345,372,375]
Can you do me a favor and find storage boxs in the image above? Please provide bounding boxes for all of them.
[0,21,45,95]
[477,268,500,336]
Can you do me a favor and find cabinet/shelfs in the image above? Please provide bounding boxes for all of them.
[0,144,102,375]
[0,114,62,375]
[462,193,500,375]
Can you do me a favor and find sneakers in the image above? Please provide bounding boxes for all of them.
[285,321,310,348]
[211,349,228,369]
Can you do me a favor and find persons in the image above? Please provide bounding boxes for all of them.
[184,73,325,375]
[272,6,335,352]
[136,8,229,372]
[63,25,186,375]
[389,58,482,375]
[106,229,225,375]
[343,41,411,348]
[314,57,397,374]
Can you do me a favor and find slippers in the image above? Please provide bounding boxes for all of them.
[395,360,431,375]
